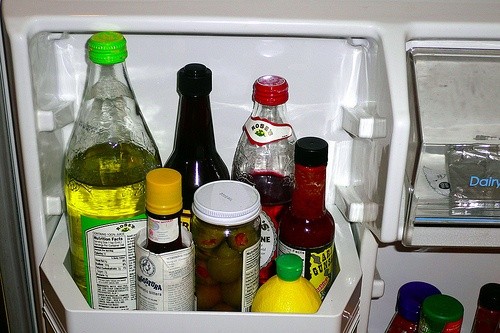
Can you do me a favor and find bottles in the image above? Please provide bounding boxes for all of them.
[384,281,442,333]
[229,73,298,285]
[163,62,231,233]
[420,294,464,333]
[470,283,500,333]
[251,253,323,313]
[60,31,163,302]
[135,169,195,311]
[277,137,335,302]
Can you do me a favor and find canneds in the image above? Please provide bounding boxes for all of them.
[191,180,261,312]
